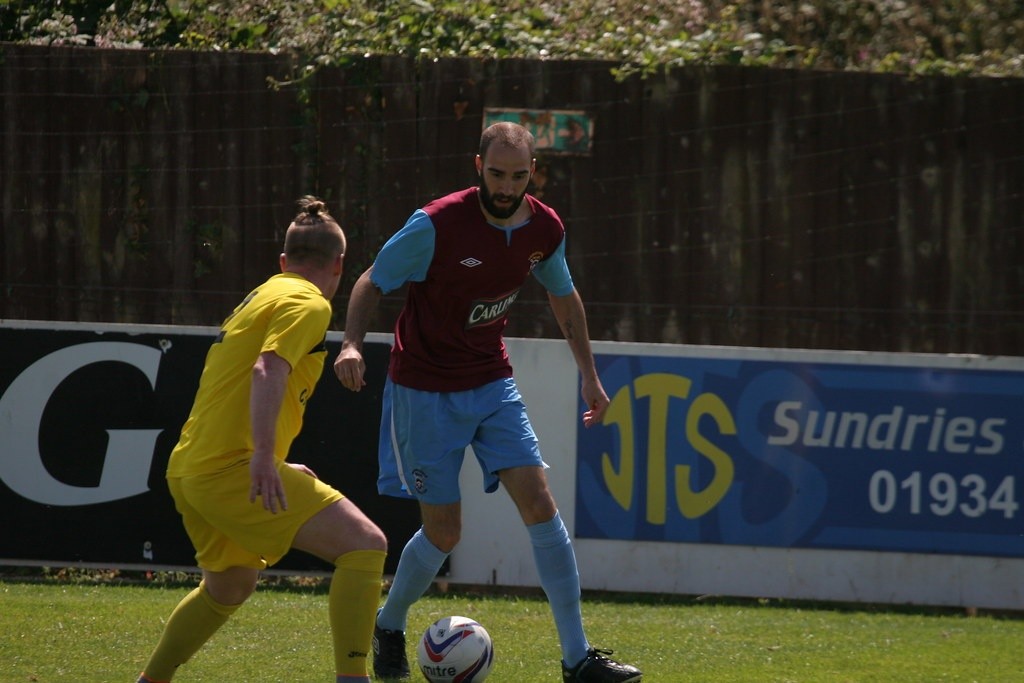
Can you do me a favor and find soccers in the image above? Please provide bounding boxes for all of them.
[416,615,495,683]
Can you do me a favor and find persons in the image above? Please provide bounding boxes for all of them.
[137,194,387,683]
[332,120,640,683]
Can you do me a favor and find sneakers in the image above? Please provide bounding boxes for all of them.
[372,608,410,680]
[560,646,644,683]
